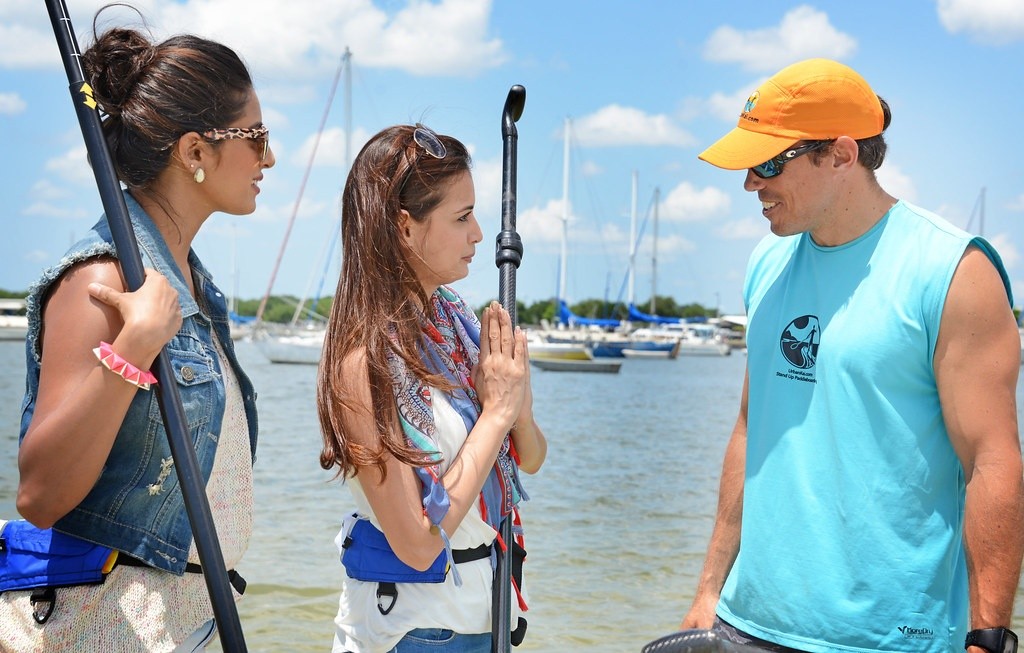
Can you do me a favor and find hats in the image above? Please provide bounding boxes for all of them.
[699,57,883,170]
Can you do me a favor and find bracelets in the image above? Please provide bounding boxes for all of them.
[92,341,158,390]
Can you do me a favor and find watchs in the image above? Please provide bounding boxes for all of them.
[964,625,1018,653]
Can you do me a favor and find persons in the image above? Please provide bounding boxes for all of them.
[679,59,1024,653]
[0,3,276,653]
[316,123,548,653]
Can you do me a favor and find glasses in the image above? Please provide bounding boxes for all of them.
[750,141,831,179]
[152,123,269,166]
[395,122,447,199]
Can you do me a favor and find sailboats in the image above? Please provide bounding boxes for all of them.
[243,44,748,372]
